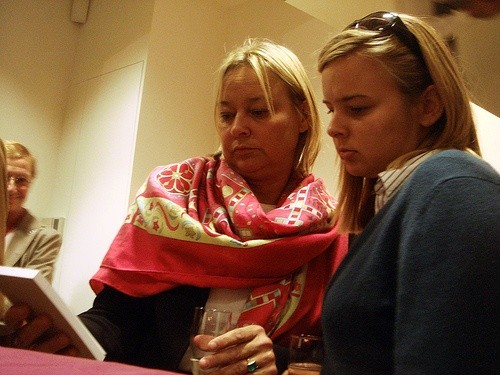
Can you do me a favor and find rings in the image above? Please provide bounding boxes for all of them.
[246,358,258,372]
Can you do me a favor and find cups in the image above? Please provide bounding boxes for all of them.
[192,307,232,375]
[288,333,324,375]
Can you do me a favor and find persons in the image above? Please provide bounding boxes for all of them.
[5,43,348,375]
[0,142,62,320]
[318,11,500,375]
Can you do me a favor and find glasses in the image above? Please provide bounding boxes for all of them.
[344,11,422,60]
[8,176,30,188]
